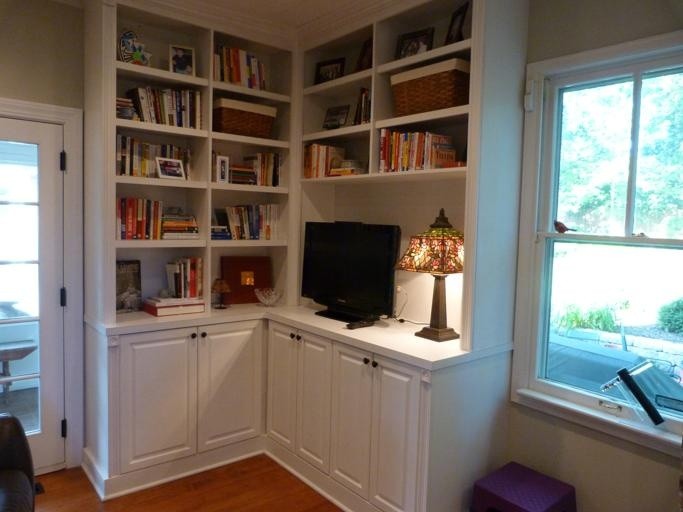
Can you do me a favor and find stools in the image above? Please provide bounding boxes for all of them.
[467,460,579,512]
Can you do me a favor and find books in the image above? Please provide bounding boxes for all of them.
[301,124,468,179]
[341,87,369,124]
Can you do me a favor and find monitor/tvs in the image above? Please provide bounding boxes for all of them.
[301,220,401,323]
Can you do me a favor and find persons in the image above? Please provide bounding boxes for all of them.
[416,41,427,55]
[404,40,416,58]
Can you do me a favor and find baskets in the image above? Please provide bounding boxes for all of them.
[211,95,280,140]
[389,58,469,118]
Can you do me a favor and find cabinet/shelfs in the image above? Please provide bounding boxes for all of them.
[82,0,533,512]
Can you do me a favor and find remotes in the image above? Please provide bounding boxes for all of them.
[347,320,375,330]
